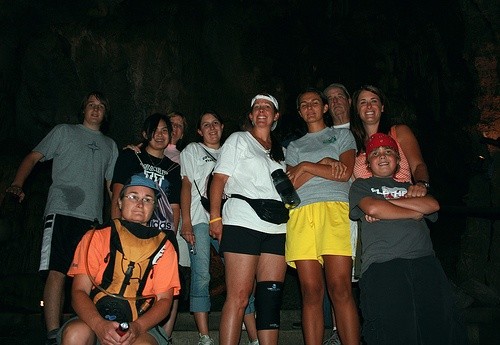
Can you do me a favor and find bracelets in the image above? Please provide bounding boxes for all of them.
[209,217,222,223]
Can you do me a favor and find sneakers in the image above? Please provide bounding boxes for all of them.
[248,339,259,345]
[197,332,216,345]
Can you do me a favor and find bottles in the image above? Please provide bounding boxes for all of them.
[271,169,301,209]
[115,322,130,336]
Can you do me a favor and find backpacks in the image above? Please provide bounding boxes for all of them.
[90,218,179,324]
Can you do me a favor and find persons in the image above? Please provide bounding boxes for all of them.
[56,175,180,344]
[180,115,262,344]
[123,112,185,166]
[324,81,369,157]
[209,92,286,345]
[4,92,120,345]
[349,134,447,345]
[285,93,361,345]
[353,86,428,199]
[111,111,180,345]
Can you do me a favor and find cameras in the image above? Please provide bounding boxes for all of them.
[190,244,196,255]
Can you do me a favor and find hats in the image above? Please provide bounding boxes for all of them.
[366,133,398,158]
[120,171,162,200]
[250,92,279,131]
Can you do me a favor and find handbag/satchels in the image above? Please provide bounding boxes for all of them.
[194,180,228,215]
[231,193,289,225]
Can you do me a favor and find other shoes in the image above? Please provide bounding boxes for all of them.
[322,330,341,345]
[292,321,302,329]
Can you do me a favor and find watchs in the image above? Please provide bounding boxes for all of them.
[416,180,430,187]
